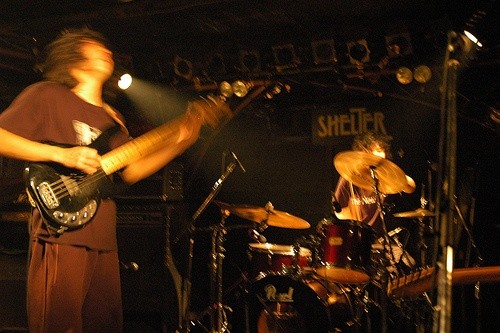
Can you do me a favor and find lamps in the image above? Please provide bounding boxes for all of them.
[396,62,432,86]
[218,79,255,98]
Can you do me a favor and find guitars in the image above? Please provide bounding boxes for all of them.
[22,92,231,230]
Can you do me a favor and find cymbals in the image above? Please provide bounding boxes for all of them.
[227,203,311,230]
[334,150,406,195]
[392,207,436,219]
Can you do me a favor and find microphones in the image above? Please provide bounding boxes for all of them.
[230,152,245,173]
[250,230,266,243]
[333,194,341,213]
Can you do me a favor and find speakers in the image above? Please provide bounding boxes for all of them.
[114,202,190,333]
[0,210,30,333]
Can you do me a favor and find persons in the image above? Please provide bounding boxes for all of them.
[0,28,216,333]
[333,131,399,229]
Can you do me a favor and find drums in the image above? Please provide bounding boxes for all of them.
[249,242,311,269]
[358,243,415,282]
[236,269,357,333]
[315,218,374,284]
